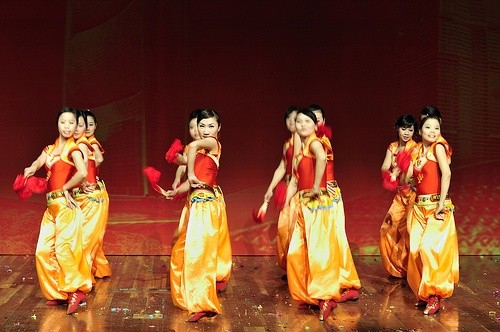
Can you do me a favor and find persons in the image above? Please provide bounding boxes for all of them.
[406,106,461,315]
[280,105,362,319]
[308,106,361,302]
[164,108,232,321]
[420,106,453,166]
[73,110,102,293]
[86,111,112,283]
[14,108,91,314]
[377,113,419,278]
[257,108,298,281]
[171,108,232,292]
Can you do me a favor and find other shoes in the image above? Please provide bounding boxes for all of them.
[423,295,441,315]
[319,300,337,320]
[46,300,65,305]
[216,281,227,292]
[335,289,359,303]
[415,299,427,308]
[67,290,86,315]
[188,311,216,322]
